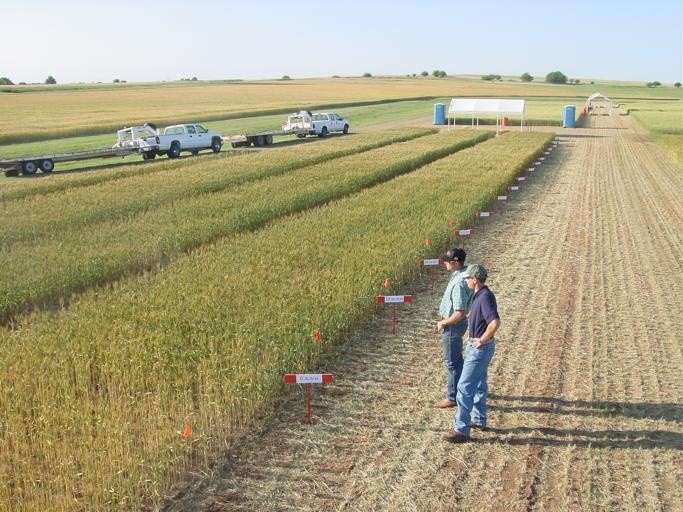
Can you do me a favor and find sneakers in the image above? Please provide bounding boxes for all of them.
[469,421,487,430]
[434,398,457,409]
[439,430,470,443]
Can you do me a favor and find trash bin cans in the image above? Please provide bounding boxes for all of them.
[434,104,445,124]
[563,105,575,128]
[502,118,508,126]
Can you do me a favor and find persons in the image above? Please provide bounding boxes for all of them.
[441,262,500,443]
[435,248,475,409]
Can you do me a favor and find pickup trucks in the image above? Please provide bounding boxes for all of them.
[288,113,349,137]
[141,124,223,160]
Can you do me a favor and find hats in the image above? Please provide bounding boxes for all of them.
[457,263,488,280]
[439,247,465,262]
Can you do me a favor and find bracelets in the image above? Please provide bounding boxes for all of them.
[440,320,445,327]
[478,338,482,344]
